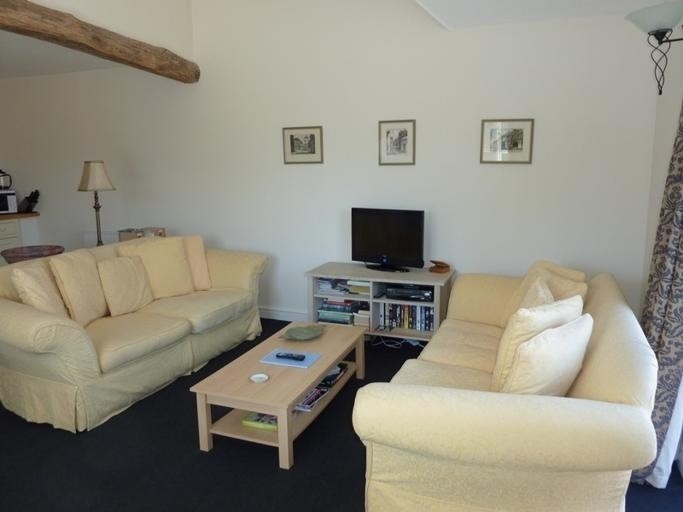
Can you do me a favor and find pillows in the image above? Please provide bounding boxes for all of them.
[510,276,556,308]
[183,234,212,290]
[494,295,583,386]
[96,255,154,317]
[12,260,71,317]
[47,246,109,327]
[118,236,196,299]
[522,260,583,291]
[501,312,594,396]
[510,268,586,304]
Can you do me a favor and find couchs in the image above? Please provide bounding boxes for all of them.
[352,273,660,512]
[0,237,270,434]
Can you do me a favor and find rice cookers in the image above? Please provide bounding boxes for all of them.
[0,169,12,188]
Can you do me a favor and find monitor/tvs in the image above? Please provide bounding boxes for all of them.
[351,206,425,273]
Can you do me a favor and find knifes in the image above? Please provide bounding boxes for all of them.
[28,189,40,203]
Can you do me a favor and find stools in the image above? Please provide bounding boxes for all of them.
[2,243,65,263]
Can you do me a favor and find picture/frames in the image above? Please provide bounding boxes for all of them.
[377,120,415,165]
[479,118,534,164]
[281,126,324,165]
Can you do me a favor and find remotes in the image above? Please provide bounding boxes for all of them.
[276,352,306,361]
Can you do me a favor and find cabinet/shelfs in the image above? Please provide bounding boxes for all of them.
[1,211,41,249]
[304,262,455,342]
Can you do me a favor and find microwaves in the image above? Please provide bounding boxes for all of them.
[0,191,18,215]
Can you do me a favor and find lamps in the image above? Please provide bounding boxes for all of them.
[76,161,116,246]
[624,1,683,95]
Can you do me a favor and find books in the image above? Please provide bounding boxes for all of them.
[317,278,434,333]
[241,363,348,430]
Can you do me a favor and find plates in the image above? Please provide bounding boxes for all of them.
[250,373,270,385]
[284,325,323,340]
